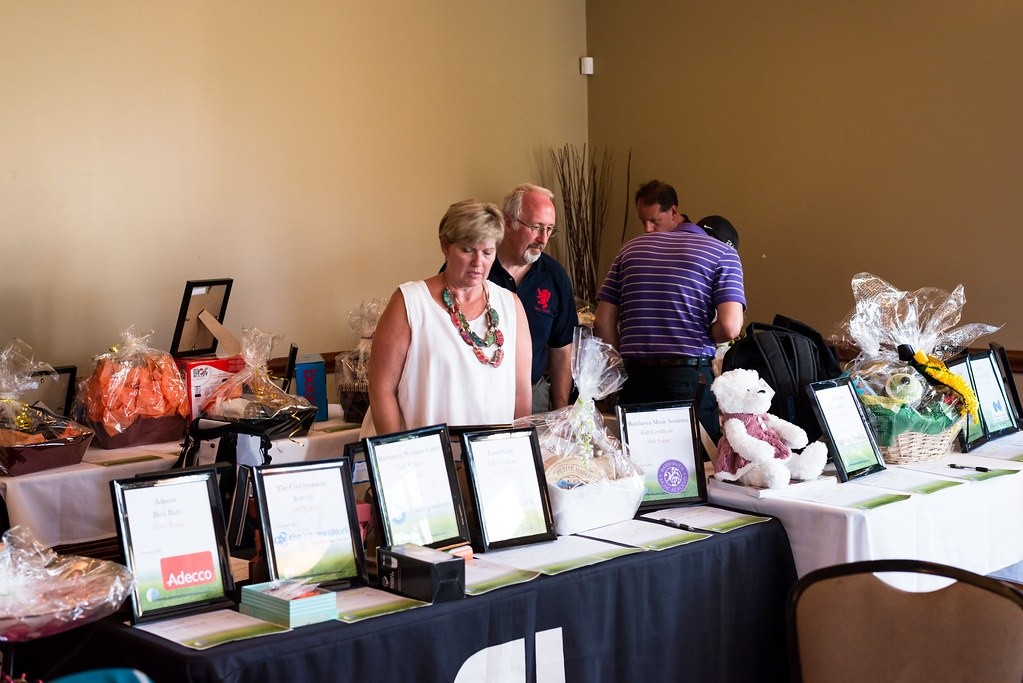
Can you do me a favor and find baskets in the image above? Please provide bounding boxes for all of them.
[860,360,965,463]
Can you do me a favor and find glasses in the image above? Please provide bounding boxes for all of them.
[514,217,559,237]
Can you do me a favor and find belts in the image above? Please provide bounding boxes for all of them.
[625,356,711,369]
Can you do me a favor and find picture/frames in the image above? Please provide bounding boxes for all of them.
[459,424,558,554]
[361,422,472,554]
[170,278,242,359]
[943,354,990,454]
[967,350,1020,442]
[614,398,707,510]
[13,363,78,419]
[248,456,370,591]
[806,376,887,484]
[108,469,239,626]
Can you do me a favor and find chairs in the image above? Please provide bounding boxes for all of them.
[785,559,1023,683]
[719,331,852,455]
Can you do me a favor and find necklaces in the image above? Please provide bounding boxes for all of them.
[442,271,504,368]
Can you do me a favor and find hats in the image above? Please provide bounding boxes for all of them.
[698,214,739,252]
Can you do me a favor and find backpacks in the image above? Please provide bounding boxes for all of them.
[722,312,842,449]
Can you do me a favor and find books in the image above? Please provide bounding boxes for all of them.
[708,475,838,498]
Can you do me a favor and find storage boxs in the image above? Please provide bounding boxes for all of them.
[175,354,244,429]
[294,352,329,423]
[375,543,466,605]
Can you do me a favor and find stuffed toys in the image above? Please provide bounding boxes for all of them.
[711,369,828,487]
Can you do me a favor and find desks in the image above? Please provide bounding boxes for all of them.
[703,429,1023,593]
[0,503,797,683]
[0,403,371,568]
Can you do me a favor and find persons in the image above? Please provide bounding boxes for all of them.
[597,181,748,462]
[439,183,579,414]
[352,199,532,505]
